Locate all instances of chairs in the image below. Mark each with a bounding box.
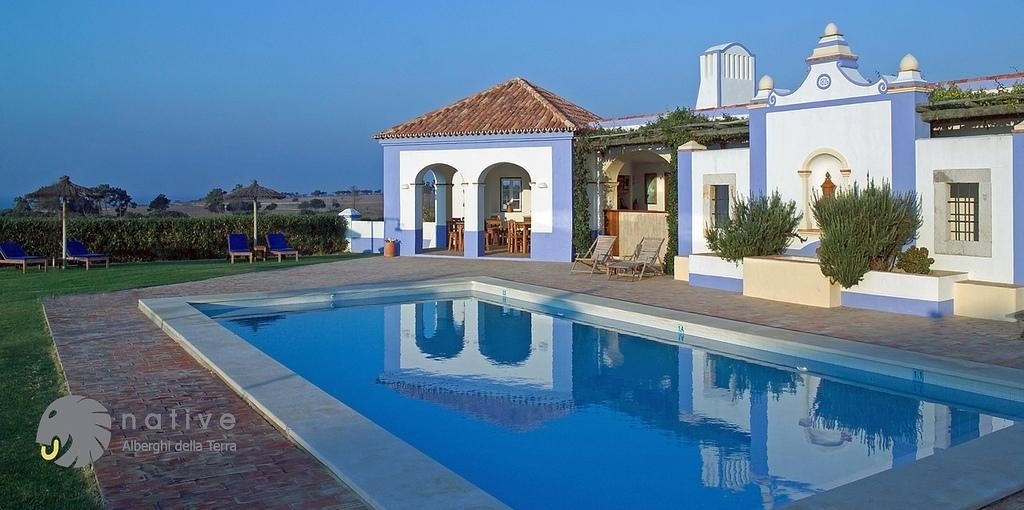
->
[607,236,666,282]
[264,232,299,263]
[568,234,618,279]
[53,238,111,271]
[0,242,51,275]
[226,233,253,264]
[447,216,530,255]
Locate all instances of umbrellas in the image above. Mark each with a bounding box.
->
[25,176,105,271]
[220,180,287,245]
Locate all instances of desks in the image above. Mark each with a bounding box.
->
[254,246,266,262]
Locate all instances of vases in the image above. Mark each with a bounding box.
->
[508,204,513,212]
[384,243,395,256]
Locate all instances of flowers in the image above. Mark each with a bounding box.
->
[385,237,397,242]
